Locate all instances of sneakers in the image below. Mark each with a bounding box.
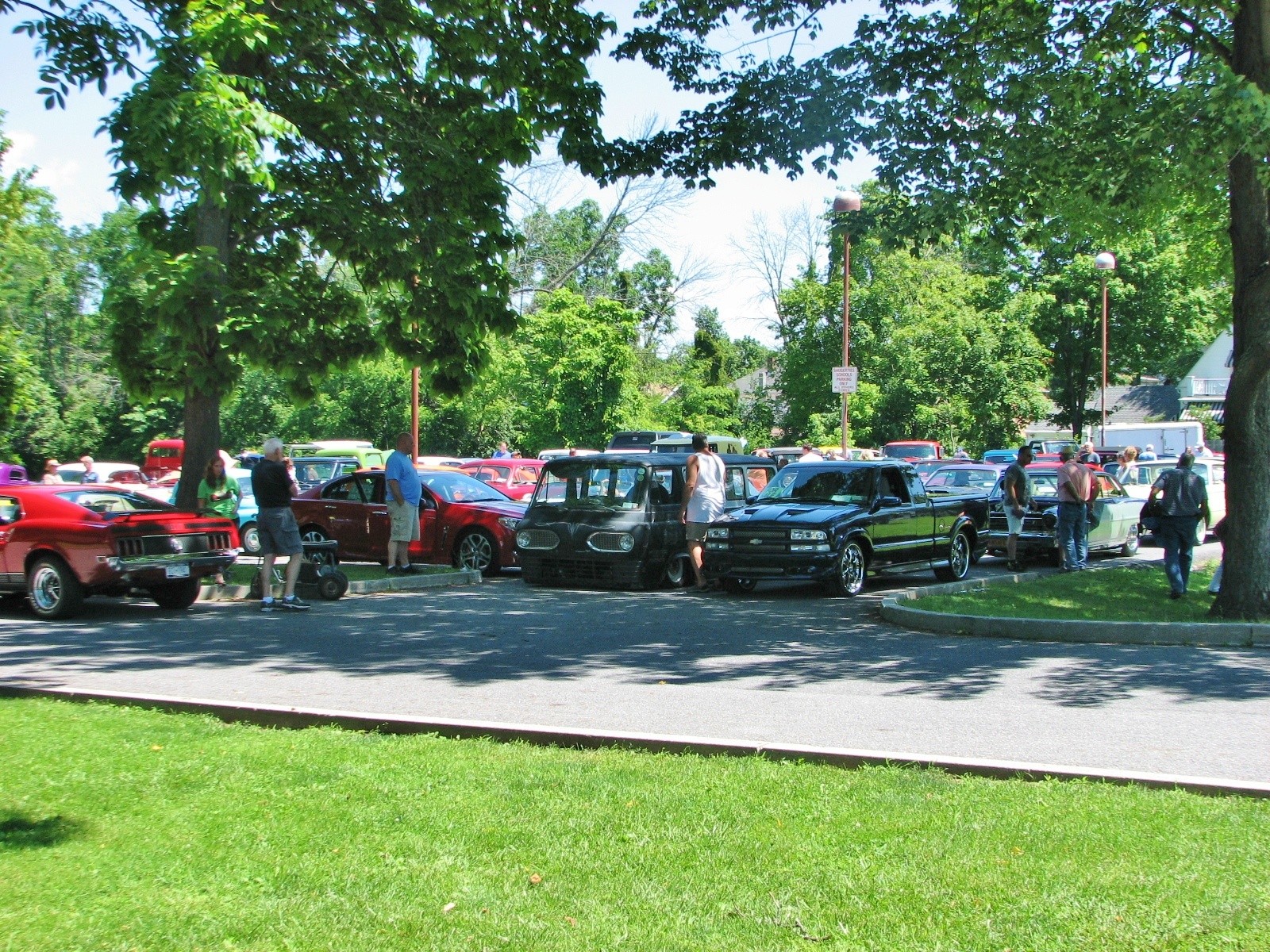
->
[214,579,226,584]
[259,598,285,612]
[1008,560,1026,572]
[282,595,310,608]
[687,584,709,593]
[401,562,423,573]
[387,566,405,576]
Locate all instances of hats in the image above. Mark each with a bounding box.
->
[1193,441,1205,448]
[1059,446,1075,454]
[45,459,62,470]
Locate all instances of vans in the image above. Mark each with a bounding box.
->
[517,452,779,591]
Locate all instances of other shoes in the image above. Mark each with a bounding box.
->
[1059,567,1071,573]
[1208,590,1220,596]
[1171,592,1181,599]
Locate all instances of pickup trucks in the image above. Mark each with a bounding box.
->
[702,459,990,600]
[880,440,962,488]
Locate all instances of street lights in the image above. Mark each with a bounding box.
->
[1095,252,1117,447]
[831,190,863,462]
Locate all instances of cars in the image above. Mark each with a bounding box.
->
[1115,455,1228,544]
[986,467,1151,566]
[923,463,1014,498]
[142,439,188,479]
[0,483,246,622]
[56,461,147,485]
[299,467,528,578]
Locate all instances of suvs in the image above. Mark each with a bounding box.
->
[0,428,877,557]
[979,439,1142,468]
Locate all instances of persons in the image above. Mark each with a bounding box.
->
[1138,444,1158,461]
[1184,446,1194,456]
[1116,446,1138,484]
[1149,451,1207,599]
[1075,442,1101,468]
[307,464,318,480]
[1004,445,1037,573]
[195,456,244,587]
[42,460,63,485]
[678,432,728,593]
[510,452,520,459]
[77,456,101,483]
[1208,515,1226,595]
[491,442,511,460]
[385,432,422,577]
[746,443,877,495]
[1193,441,1214,459]
[1056,445,1101,572]
[251,438,311,613]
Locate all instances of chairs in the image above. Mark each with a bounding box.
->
[627,469,667,508]
[865,476,894,507]
[334,490,348,499]
[810,477,833,501]
